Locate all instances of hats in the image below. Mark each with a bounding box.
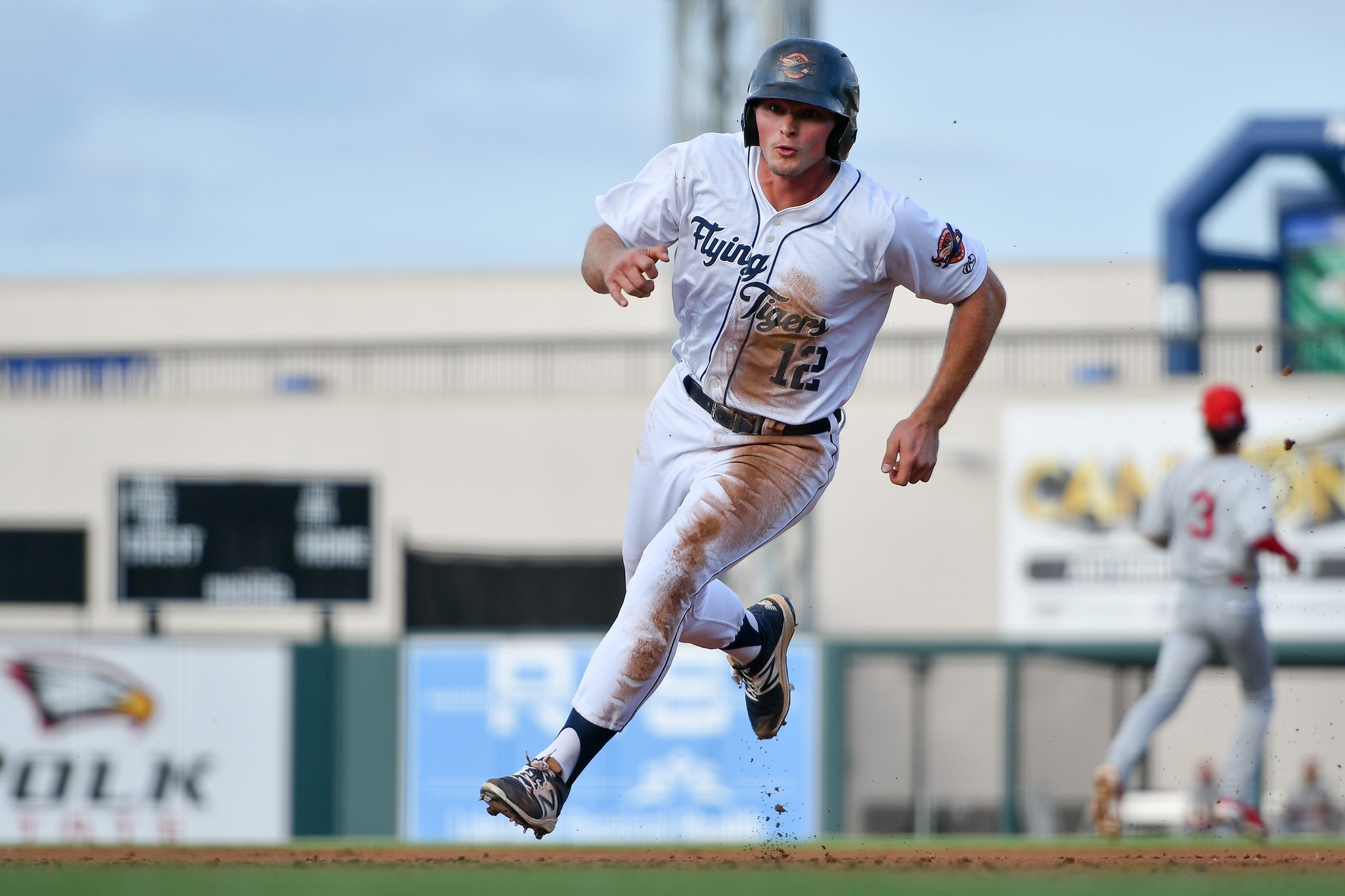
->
[1203,386,1243,429]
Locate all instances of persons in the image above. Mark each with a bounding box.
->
[1090,384,1299,846]
[1282,762,1336,833]
[479,36,1007,840]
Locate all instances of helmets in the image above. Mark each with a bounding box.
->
[741,37,860,162]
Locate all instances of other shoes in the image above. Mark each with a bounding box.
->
[1211,797,1266,843]
[1094,762,1124,836]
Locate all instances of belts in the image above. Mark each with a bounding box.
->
[682,375,842,436]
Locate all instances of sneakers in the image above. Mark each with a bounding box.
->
[730,595,798,738]
[479,748,567,840]
